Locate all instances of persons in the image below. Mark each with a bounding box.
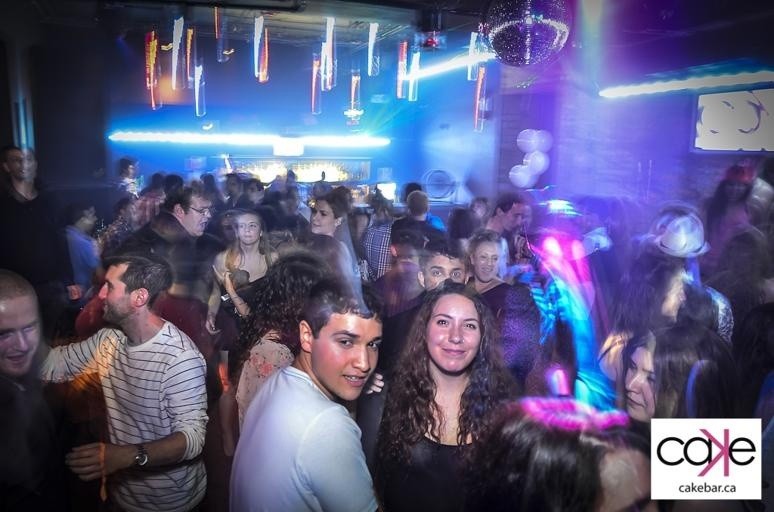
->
[0,147,772,512]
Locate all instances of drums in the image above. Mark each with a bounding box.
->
[421,169,453,199]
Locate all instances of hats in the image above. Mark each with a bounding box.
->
[653,204,712,258]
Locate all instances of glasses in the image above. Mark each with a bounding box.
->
[188,206,218,216]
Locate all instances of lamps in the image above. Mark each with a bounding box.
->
[485,1,575,69]
